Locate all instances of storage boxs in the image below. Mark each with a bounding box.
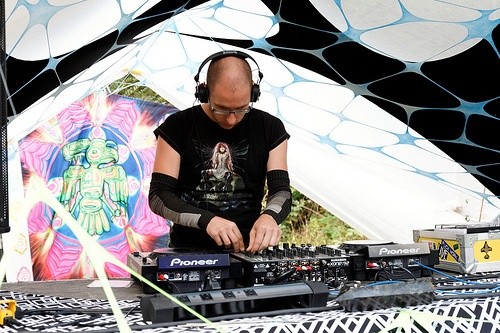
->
[413,224,500,273]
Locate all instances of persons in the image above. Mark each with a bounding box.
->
[148,57,292,252]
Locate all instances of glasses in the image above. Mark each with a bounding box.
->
[208,98,253,115]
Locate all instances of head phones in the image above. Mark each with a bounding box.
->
[193,50,263,104]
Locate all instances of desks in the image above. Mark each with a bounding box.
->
[0,270,500,333]
